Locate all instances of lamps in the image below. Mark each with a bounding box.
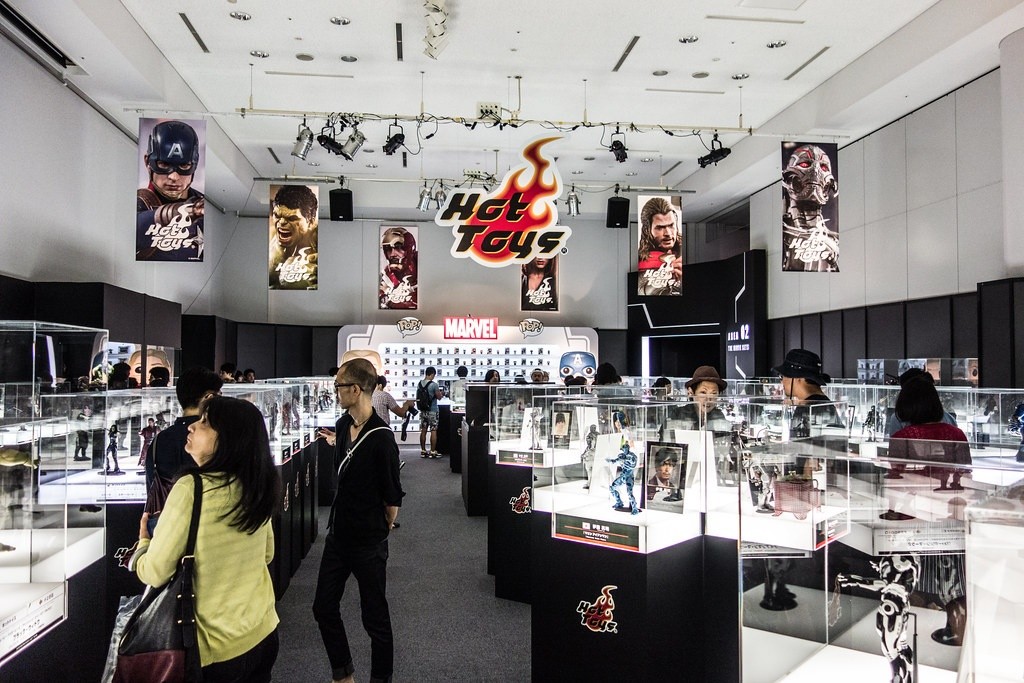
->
[292,115,731,216]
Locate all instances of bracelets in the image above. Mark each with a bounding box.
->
[332,438,335,445]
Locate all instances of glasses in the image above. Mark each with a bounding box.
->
[334,381,365,392]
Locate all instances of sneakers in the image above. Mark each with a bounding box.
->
[421,449,430,457]
[429,451,442,458]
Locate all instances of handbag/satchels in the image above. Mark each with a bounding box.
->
[142,475,175,538]
[100,470,206,683]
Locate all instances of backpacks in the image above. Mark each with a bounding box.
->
[416,380,436,410]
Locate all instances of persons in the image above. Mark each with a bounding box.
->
[0,407,166,553]
[416,367,445,458]
[591,362,621,392]
[651,378,671,400]
[451,366,469,403]
[129,396,282,683]
[138,121,204,262]
[563,375,589,393]
[605,444,639,516]
[381,228,416,310]
[521,258,557,311]
[555,414,567,434]
[771,349,846,475]
[268,388,334,442]
[314,359,407,683]
[372,376,415,470]
[530,368,544,381]
[146,366,224,511]
[527,409,543,449]
[580,425,600,490]
[637,197,683,295]
[219,362,254,383]
[648,448,677,499]
[887,367,973,518]
[108,362,168,389]
[613,413,622,433]
[837,552,921,683]
[659,366,727,442]
[269,184,318,290]
[862,406,880,442]
[485,370,500,382]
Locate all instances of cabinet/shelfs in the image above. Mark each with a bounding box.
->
[0,321,1024,682]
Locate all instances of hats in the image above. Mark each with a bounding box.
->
[685,366,727,392]
[772,348,827,386]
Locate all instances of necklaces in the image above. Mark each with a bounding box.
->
[354,419,368,428]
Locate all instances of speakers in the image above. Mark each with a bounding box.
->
[329,189,354,221]
[607,198,629,229]
